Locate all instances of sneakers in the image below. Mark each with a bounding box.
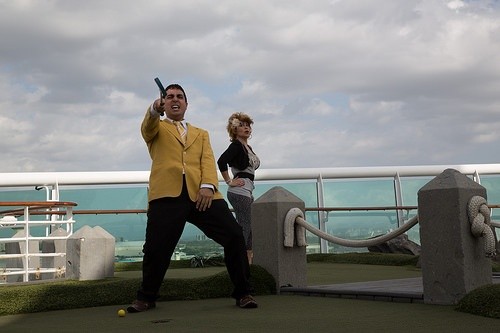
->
[236,293,257,307]
[127,299,154,312]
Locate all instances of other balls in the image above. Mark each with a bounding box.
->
[118,310,125,317]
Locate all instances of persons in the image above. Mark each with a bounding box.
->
[126,84,258,313]
[217,112,260,263]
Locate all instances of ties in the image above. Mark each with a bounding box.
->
[174,121,188,143]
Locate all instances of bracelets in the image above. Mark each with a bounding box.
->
[227,180,231,184]
